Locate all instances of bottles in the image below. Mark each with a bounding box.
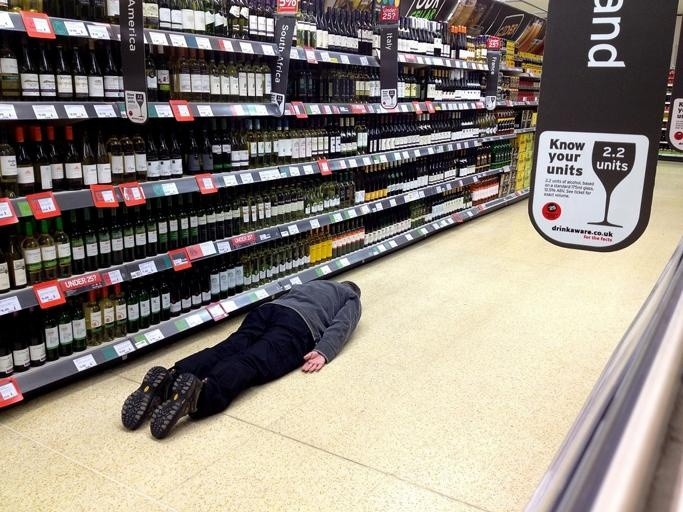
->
[0,0,517,377]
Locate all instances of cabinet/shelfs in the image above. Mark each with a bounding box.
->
[0,0,541,401]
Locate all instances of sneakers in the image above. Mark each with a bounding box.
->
[121,365,171,430]
[150,373,203,439]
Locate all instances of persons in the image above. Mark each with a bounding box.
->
[122,280,364,438]
